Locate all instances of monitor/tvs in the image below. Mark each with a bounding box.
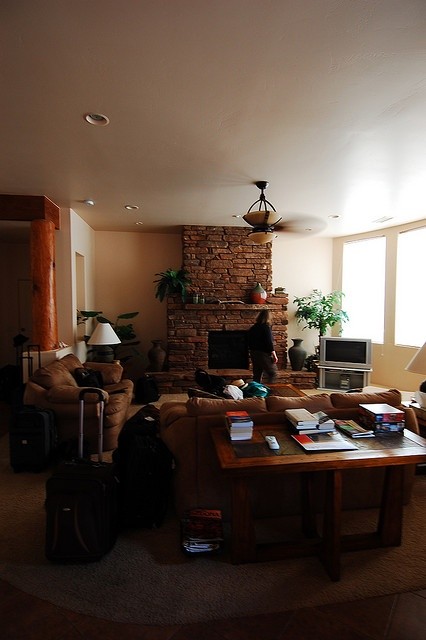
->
[320,336,372,369]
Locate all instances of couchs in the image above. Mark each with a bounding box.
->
[155,390,420,519]
[23,353,134,451]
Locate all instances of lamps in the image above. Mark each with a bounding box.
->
[87,322,122,362]
[243,181,279,245]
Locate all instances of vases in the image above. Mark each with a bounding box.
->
[148,339,167,369]
[252,282,268,303]
[289,339,307,371]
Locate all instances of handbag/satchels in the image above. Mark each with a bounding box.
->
[134,373,161,402]
[194,368,231,398]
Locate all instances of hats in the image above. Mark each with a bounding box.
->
[12,333,29,347]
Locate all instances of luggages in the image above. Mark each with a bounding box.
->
[44,387,119,565]
[0,364,24,404]
[9,357,55,474]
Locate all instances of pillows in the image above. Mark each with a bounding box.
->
[187,388,222,399]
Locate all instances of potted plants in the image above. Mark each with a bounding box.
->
[154,268,192,302]
[295,290,349,371]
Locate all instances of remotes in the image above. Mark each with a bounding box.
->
[265,435,280,450]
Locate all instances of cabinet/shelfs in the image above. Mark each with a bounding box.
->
[316,366,370,393]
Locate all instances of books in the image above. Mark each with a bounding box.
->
[335,403,405,438]
[224,410,254,441]
[285,408,335,435]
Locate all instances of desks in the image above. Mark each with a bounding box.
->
[207,426,425,582]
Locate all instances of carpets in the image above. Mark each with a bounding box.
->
[1,439,425,626]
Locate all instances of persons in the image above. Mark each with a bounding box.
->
[249,310,278,384]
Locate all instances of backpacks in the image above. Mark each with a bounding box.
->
[112,404,177,538]
